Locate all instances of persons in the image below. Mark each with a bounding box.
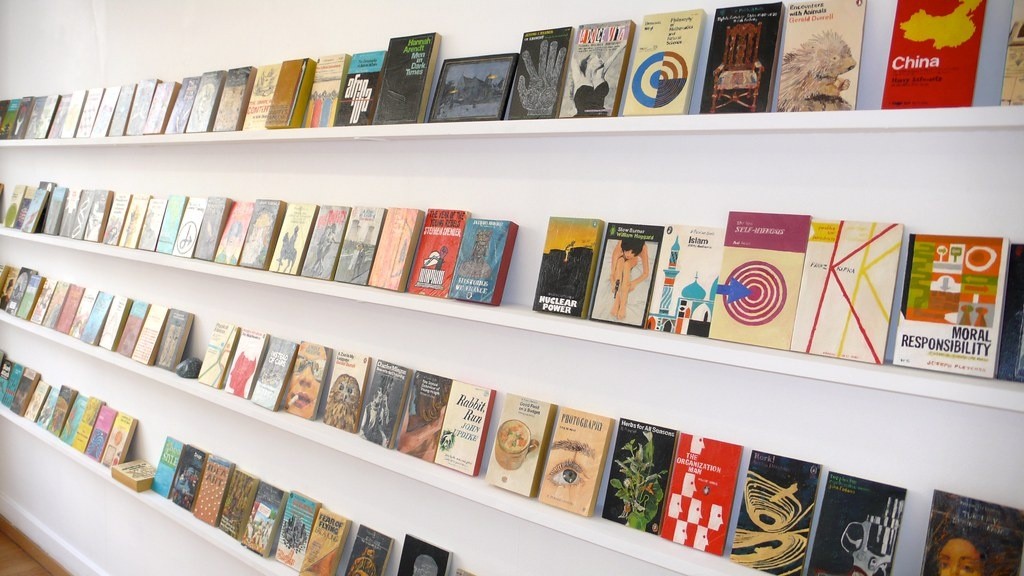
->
[397,371,453,463]
[937,530,996,576]
[285,342,326,419]
[312,223,339,275]
[610,238,649,319]
[353,245,365,277]
[360,376,389,448]
[570,40,626,117]
[0,279,13,311]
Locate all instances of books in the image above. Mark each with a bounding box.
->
[0,181,1024,576]
[0,0,1024,140]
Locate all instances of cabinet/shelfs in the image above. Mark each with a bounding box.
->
[0,105,1024,575]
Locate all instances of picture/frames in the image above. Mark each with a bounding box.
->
[426,52,520,122]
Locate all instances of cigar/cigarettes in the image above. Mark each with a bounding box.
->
[285,396,299,409]
